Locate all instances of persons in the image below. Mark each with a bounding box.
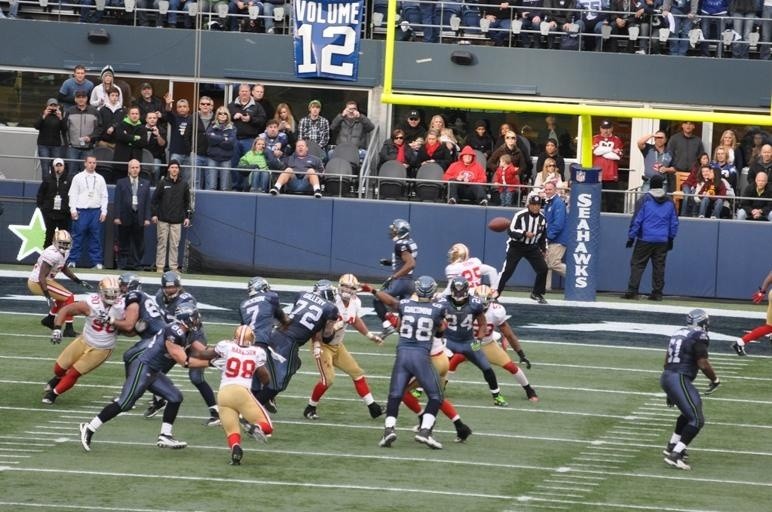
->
[3,0,772,469]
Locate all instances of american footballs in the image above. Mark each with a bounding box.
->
[488,217,511,232]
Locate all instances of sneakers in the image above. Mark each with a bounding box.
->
[42,376,61,404]
[208,396,278,444]
[229,444,243,464]
[370,405,387,419]
[662,442,692,471]
[379,426,396,447]
[144,399,168,419]
[78,420,96,453]
[493,394,509,407]
[618,291,664,301]
[303,407,319,419]
[156,434,188,450]
[448,197,456,204]
[528,291,547,305]
[527,395,539,402]
[313,189,322,197]
[729,340,749,356]
[270,186,281,195]
[41,316,81,337]
[411,389,422,398]
[480,199,488,206]
[415,409,471,448]
[67,261,182,276]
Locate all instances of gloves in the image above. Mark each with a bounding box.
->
[311,348,323,361]
[51,326,64,345]
[752,286,766,304]
[470,338,482,353]
[377,258,390,269]
[380,276,392,292]
[666,396,675,408]
[47,296,58,308]
[358,282,374,293]
[518,357,531,369]
[668,239,673,250]
[370,335,383,346]
[97,309,116,326]
[625,237,634,248]
[703,377,720,395]
[80,280,94,289]
[209,356,227,372]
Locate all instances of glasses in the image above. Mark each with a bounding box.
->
[200,102,212,106]
[218,112,228,116]
[394,136,404,140]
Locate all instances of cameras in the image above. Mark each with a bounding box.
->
[51,109,56,112]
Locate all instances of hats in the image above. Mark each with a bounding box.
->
[101,65,115,78]
[76,89,88,98]
[407,110,420,120]
[53,158,65,167]
[47,98,58,107]
[528,195,542,205]
[598,119,613,130]
[307,100,322,109]
[650,175,665,184]
[141,82,152,90]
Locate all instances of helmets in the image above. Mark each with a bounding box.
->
[97,273,143,305]
[234,325,256,347]
[248,276,271,297]
[450,276,492,311]
[162,271,182,300]
[414,275,438,298]
[175,302,202,334]
[684,308,711,332]
[53,229,73,255]
[389,218,411,240]
[314,273,360,302]
[446,243,469,264]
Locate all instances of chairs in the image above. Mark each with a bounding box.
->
[302,136,530,207]
[93,146,154,186]
[674,172,689,217]
